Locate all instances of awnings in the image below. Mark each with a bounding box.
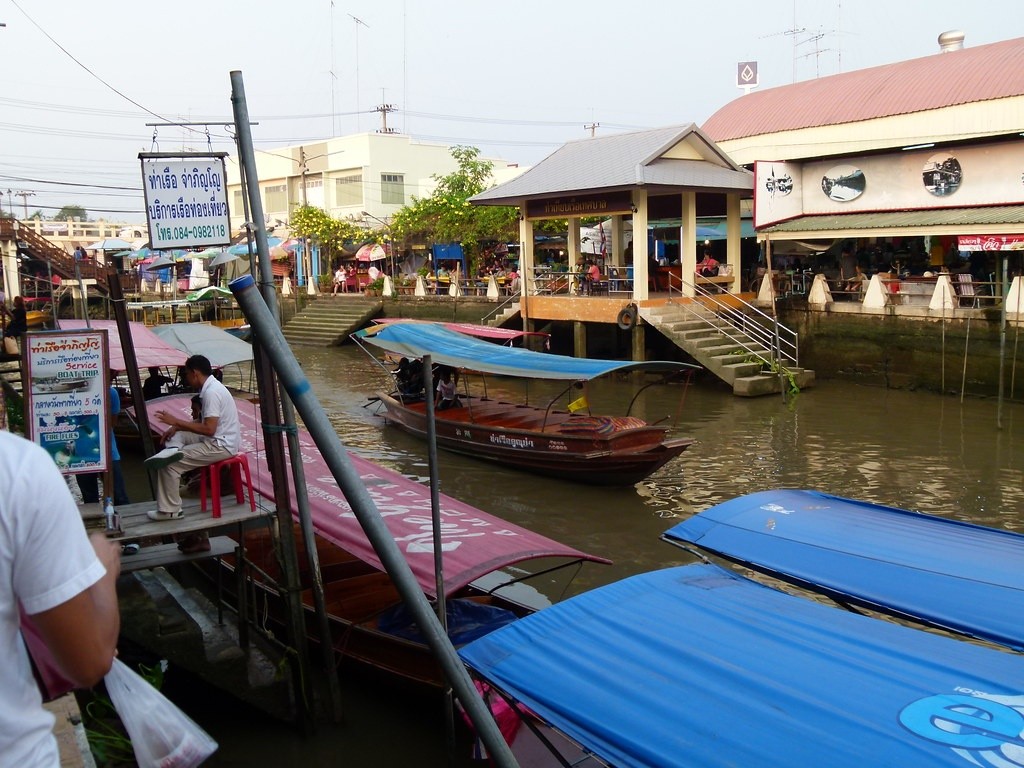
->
[681,218,757,240]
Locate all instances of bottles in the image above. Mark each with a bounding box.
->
[105,497,115,530]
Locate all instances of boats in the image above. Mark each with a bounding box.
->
[347,316,701,488]
[661,487,1024,656]
[204,317,252,341]
[0,297,53,328]
[463,563,1023,767]
[56,318,258,395]
[132,392,618,695]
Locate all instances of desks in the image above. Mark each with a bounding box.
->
[483,277,512,284]
[905,275,954,282]
[430,276,451,283]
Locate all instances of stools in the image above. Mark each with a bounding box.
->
[201,451,256,518]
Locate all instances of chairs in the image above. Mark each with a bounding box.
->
[954,274,981,309]
[569,265,657,299]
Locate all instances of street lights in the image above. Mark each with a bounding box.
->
[0,189,13,217]
[15,189,37,220]
[274,218,308,291]
[362,210,396,292]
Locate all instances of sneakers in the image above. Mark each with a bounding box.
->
[144,448,184,469]
[146,508,185,521]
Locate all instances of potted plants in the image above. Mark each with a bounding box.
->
[277,256,291,276]
[319,274,334,293]
[364,275,417,296]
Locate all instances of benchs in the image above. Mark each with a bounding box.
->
[407,399,585,433]
[218,524,493,630]
[697,276,735,294]
[212,318,244,328]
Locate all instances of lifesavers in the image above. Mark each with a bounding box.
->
[626,303,637,315]
[618,308,636,330]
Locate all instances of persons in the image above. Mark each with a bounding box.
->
[333,260,519,296]
[0,429,122,768]
[575,240,720,295]
[0,296,28,344]
[390,357,463,411]
[74,246,87,260]
[144,355,240,520]
[792,250,868,302]
[75,369,130,506]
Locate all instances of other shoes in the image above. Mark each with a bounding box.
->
[178,530,210,554]
[455,399,463,408]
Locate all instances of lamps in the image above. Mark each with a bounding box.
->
[630,201,637,213]
[516,211,523,221]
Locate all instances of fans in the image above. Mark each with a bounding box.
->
[890,255,906,276]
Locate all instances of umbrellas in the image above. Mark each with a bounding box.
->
[84,236,298,271]
[356,243,395,273]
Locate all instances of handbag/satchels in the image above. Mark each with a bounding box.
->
[101,658,219,768]
